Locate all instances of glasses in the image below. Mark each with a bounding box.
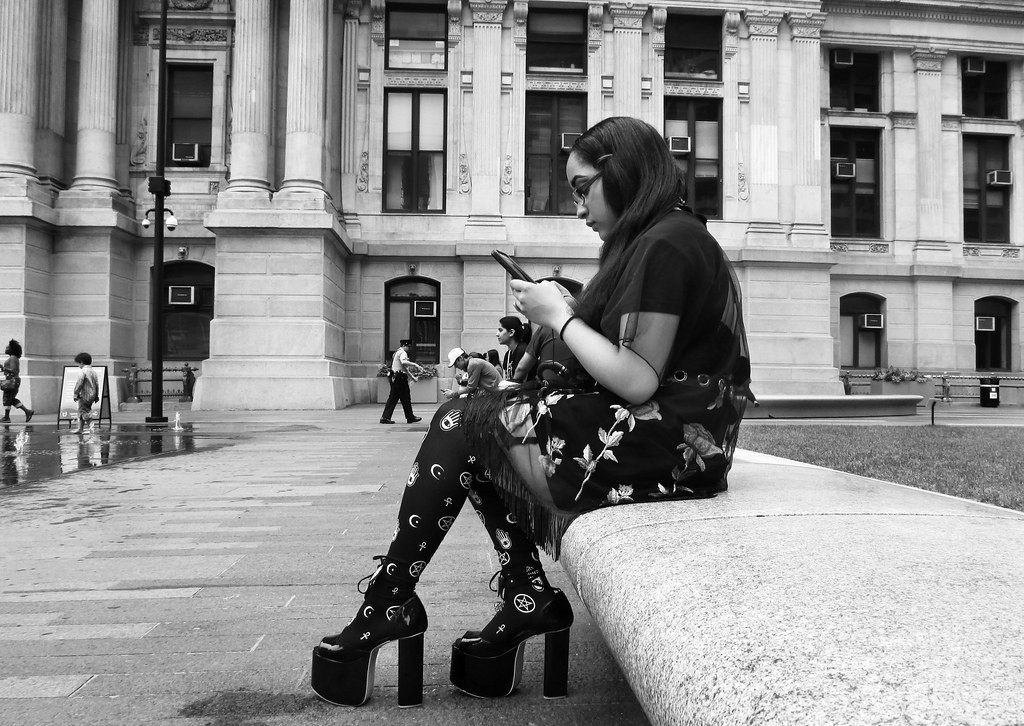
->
[571,169,604,207]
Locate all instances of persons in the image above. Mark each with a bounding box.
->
[442,347,503,399]
[73,352,100,435]
[0,339,35,423]
[380,339,423,424]
[513,281,584,385]
[311,116,759,709]
[496,316,532,383]
[456,352,479,398]
[487,350,503,379]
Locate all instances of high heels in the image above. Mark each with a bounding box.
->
[310,568,428,710]
[449,567,574,699]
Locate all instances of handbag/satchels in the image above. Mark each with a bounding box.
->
[0,370,21,391]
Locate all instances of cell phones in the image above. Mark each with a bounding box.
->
[440,389,445,392]
[491,249,535,285]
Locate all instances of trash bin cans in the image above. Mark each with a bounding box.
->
[980,378,999,407]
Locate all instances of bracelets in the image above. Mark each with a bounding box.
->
[560,316,575,340]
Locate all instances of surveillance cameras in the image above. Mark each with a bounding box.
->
[142,219,151,229]
[166,216,178,231]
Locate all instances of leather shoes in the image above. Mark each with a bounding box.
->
[380,415,395,424]
[407,416,423,423]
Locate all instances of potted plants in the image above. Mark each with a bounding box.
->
[871,365,936,406]
[377,361,438,404]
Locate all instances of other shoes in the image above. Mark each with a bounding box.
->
[0,416,11,423]
[26,409,34,422]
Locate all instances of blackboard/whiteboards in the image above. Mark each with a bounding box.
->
[59,366,111,420]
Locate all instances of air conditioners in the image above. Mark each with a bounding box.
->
[666,136,692,156]
[414,300,438,317]
[558,132,584,154]
[172,143,203,162]
[975,316,995,332]
[858,314,884,329]
[986,170,1013,187]
[834,49,853,68]
[832,163,856,180]
[168,285,201,305]
[963,57,986,77]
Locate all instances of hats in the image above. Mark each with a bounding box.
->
[399,339,413,347]
[447,346,463,368]
